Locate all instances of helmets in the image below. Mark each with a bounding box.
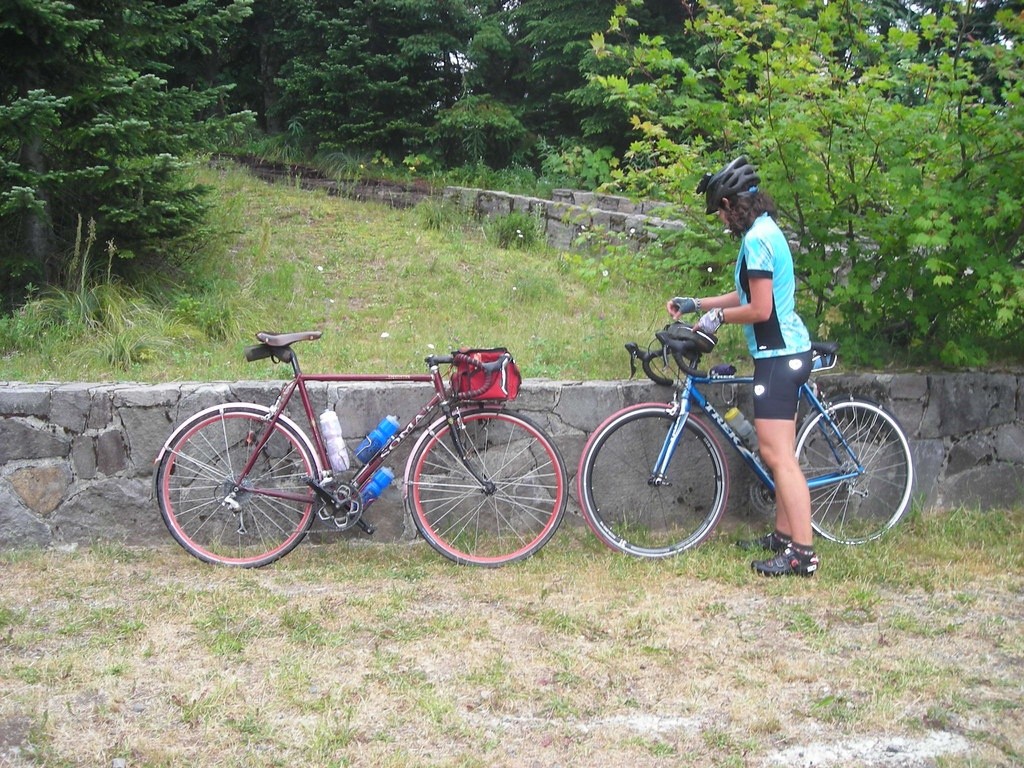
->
[706,155,762,215]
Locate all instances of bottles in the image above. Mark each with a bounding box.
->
[349,466,396,515]
[319,408,350,473]
[354,414,401,462]
[725,407,758,452]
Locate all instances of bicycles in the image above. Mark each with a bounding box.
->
[154,330,570,570]
[575,331,915,559]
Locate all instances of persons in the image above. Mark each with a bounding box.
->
[666,155,818,579]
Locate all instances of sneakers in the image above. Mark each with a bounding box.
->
[751,542,817,578]
[734,530,794,555]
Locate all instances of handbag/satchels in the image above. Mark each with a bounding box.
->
[448,349,520,401]
